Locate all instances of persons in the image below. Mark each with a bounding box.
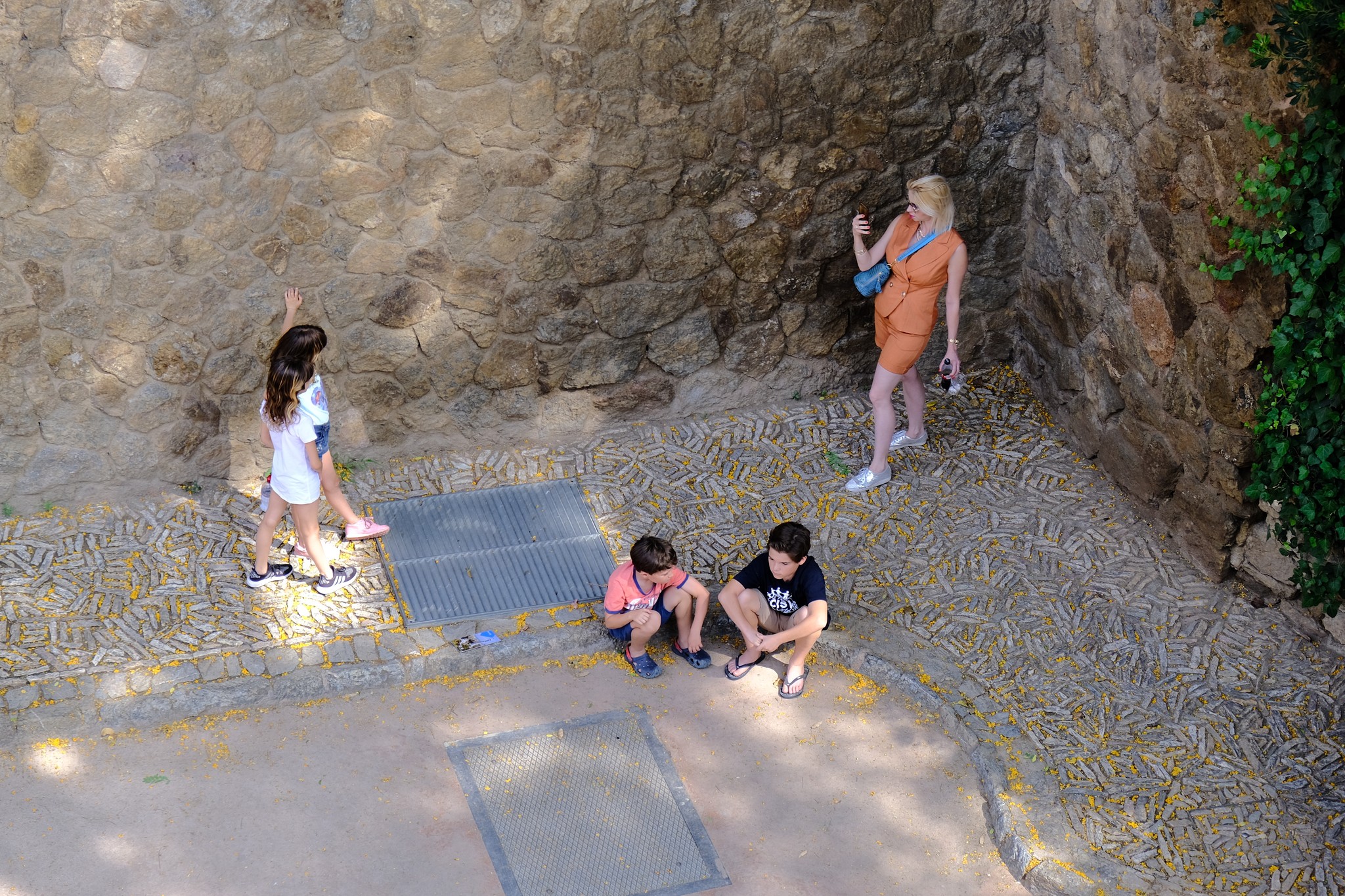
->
[263,287,389,559]
[845,175,969,492]
[247,356,357,594]
[604,535,711,678]
[718,521,831,699]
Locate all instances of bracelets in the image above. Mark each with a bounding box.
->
[853,243,866,255]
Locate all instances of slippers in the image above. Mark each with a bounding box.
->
[671,638,711,669]
[624,643,662,678]
[725,651,765,680]
[779,665,809,699]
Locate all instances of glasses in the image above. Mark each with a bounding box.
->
[905,194,920,214]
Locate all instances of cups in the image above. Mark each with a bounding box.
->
[949,372,967,395]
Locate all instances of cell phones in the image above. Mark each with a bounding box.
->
[859,202,869,235]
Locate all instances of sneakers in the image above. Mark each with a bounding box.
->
[888,430,929,450]
[345,516,390,540]
[844,465,892,492]
[294,540,312,559]
[246,562,293,587]
[316,565,357,594]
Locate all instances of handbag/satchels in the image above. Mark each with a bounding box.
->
[853,261,892,298]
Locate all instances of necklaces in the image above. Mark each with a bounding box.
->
[916,225,932,244]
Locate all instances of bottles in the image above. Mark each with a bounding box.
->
[259,475,272,512]
[941,358,953,391]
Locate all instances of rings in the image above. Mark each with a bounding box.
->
[856,226,858,229]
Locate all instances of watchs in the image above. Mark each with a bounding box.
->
[947,339,959,345]
